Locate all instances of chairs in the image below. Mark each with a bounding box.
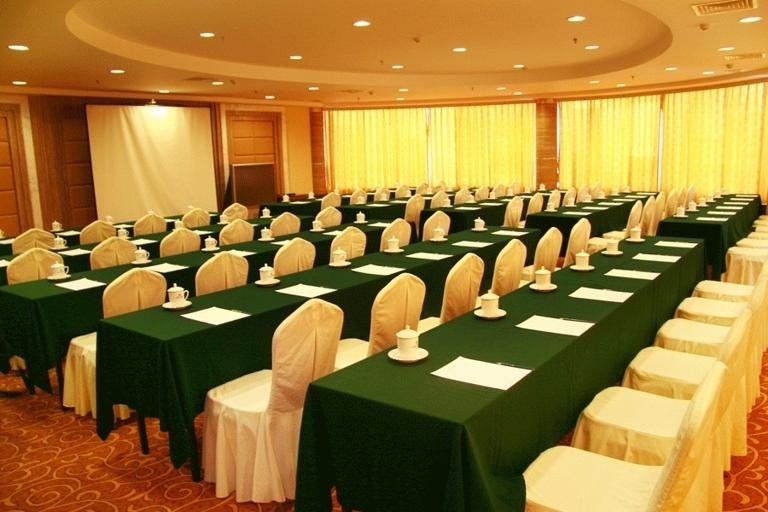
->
[0,181,768,512]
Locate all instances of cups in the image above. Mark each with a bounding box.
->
[534,265,552,288]
[606,238,620,252]
[479,290,501,314]
[395,325,420,357]
[167,282,189,305]
[575,248,590,268]
[442,182,728,217]
[630,226,642,240]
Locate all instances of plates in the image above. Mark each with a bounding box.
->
[625,237,647,243]
[568,264,596,272]
[531,282,557,292]
[254,279,280,286]
[602,250,625,256]
[388,348,430,364]
[162,300,192,310]
[474,309,509,320]
